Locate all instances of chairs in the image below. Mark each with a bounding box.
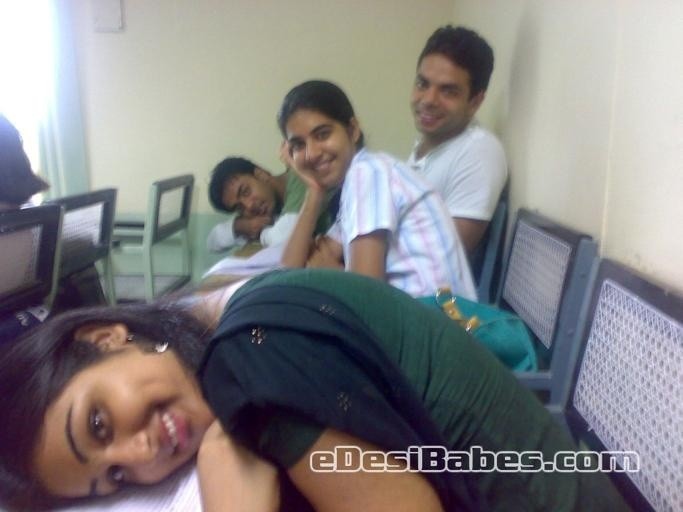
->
[477,209,598,405]
[1,206,72,342]
[111,174,194,306]
[471,199,506,305]
[514,259,683,512]
[48,188,116,314]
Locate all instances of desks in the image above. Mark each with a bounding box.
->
[0,238,264,512]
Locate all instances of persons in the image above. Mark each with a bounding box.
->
[0,116,78,346]
[206,157,337,248]
[0,264,584,512]
[276,79,479,304]
[405,23,508,265]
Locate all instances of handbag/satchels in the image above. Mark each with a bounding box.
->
[415,287,538,373]
